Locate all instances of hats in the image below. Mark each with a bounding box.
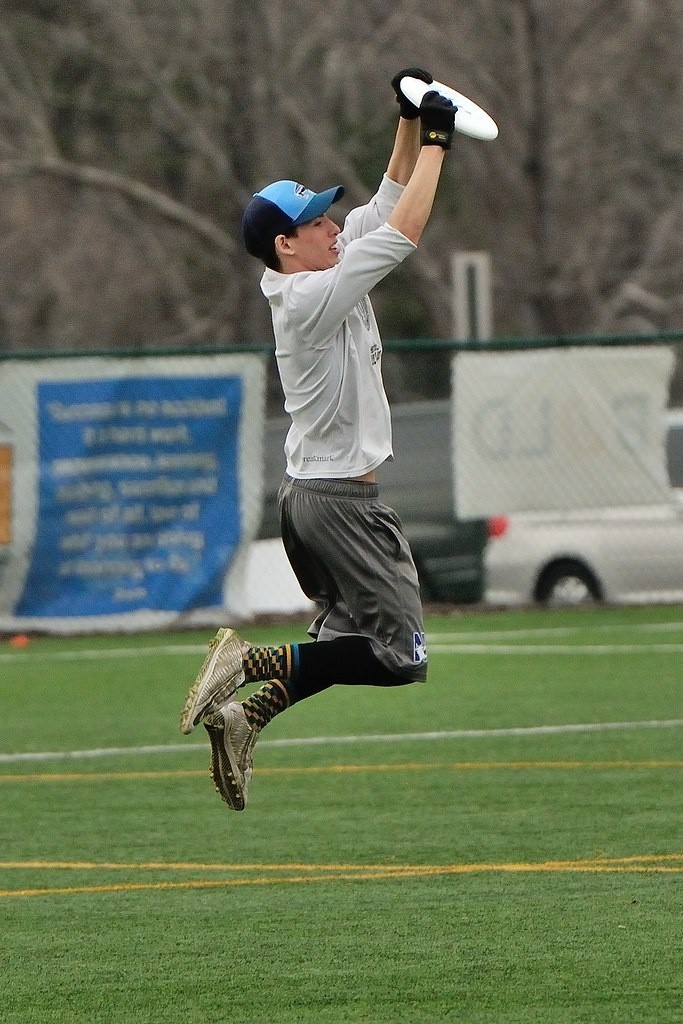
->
[242,179,344,258]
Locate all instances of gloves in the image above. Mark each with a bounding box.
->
[419,90,458,150]
[391,67,434,120]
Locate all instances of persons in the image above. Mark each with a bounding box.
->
[177,69,458,811]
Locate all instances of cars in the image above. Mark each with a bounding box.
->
[255,484,509,606]
[481,406,683,608]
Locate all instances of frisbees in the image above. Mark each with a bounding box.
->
[399,75,500,142]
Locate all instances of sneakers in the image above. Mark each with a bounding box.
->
[203,700,259,811]
[180,627,252,734]
